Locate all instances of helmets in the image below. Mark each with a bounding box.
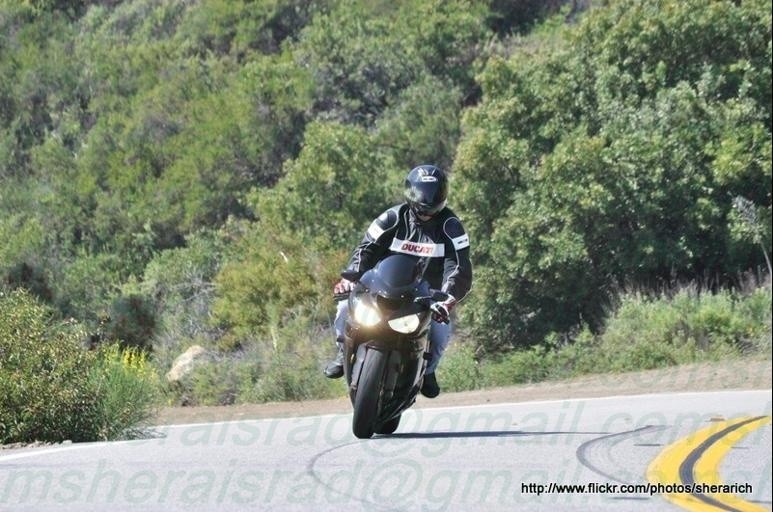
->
[404,165,448,218]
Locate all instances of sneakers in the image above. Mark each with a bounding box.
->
[324,363,344,379]
[421,372,440,398]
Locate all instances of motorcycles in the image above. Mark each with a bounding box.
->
[333,255,450,439]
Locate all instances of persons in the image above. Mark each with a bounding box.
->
[323,164,472,397]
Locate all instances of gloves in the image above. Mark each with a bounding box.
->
[333,278,355,294]
[430,294,457,324]
